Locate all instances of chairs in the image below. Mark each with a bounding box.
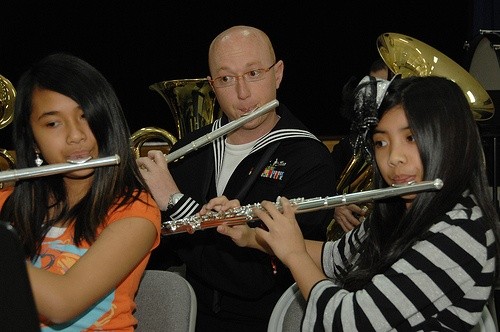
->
[268,280,496,332]
[132,270,197,332]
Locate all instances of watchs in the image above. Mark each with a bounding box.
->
[167,193,184,209]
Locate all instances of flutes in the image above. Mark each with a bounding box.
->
[160,178,445,233]
[141,100,278,174]
[0,154,121,183]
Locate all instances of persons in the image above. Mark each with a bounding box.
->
[328,53,394,234]
[0,54,161,332]
[135,26,336,332]
[199,75,500,332]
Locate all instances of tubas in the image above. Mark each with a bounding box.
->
[325,32,496,240]
[126,77,217,190]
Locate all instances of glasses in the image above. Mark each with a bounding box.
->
[210,61,277,89]
